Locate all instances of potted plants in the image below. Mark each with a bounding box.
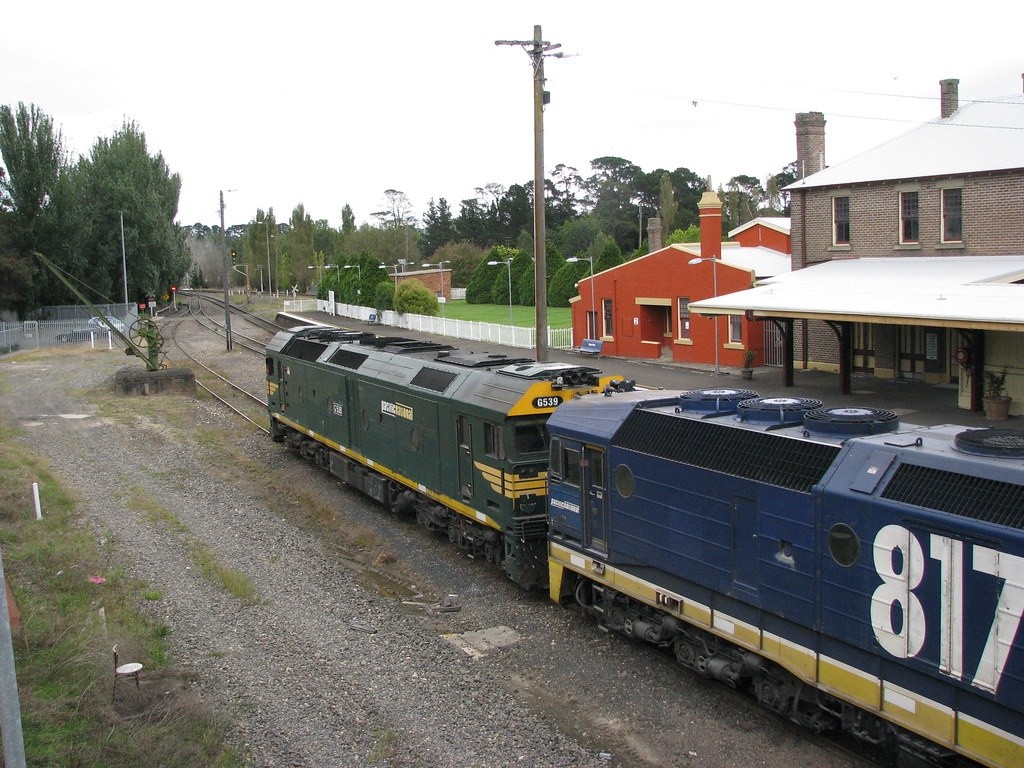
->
[741,348,756,379]
[982,365,1012,421]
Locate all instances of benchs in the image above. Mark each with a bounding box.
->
[363,314,376,326]
[574,338,603,360]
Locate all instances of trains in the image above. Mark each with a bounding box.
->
[263,323,1024,768]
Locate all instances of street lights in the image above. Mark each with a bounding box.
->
[378,265,398,293]
[487,256,513,325]
[565,256,596,341]
[118,210,128,304]
[307,265,322,289]
[344,265,360,281]
[421,261,451,318]
[688,255,722,375]
[325,265,340,283]
[257,222,271,297]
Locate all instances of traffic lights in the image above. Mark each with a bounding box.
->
[232,249,236,258]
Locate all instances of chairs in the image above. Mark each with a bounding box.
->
[111,644,143,703]
